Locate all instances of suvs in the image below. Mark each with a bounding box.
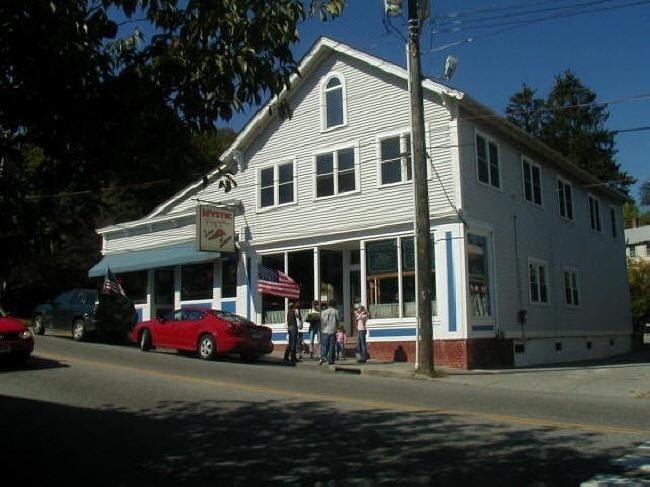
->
[32,288,138,343]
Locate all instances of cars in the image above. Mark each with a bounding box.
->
[128,308,273,360]
[0,306,35,359]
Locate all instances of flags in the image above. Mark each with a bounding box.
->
[257,261,301,300]
[103,267,125,295]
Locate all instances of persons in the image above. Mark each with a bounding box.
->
[319,301,327,310]
[306,301,321,358]
[319,300,340,364]
[354,307,371,362]
[336,325,347,360]
[284,299,301,362]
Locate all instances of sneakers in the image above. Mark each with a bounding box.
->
[283,357,304,363]
